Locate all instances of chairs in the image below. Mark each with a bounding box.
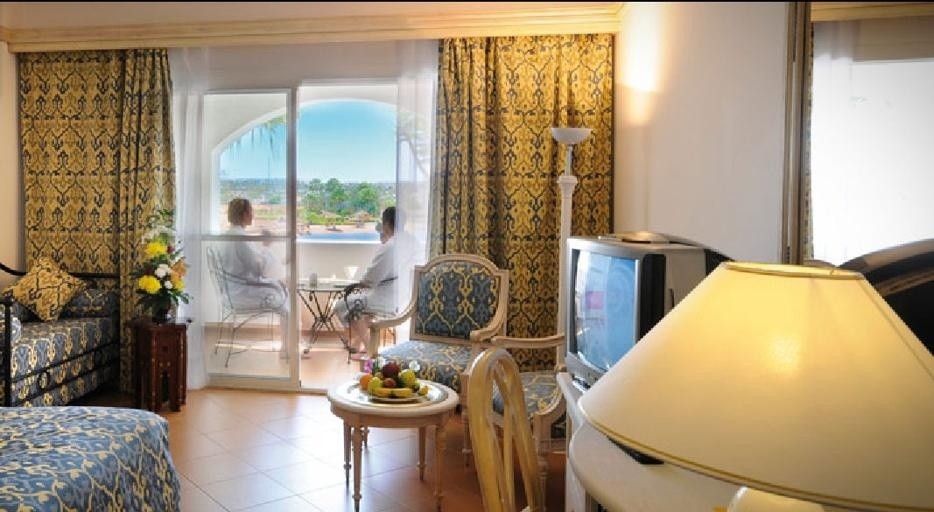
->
[467,349,543,512]
[367,253,510,467]
[344,275,399,365]
[491,332,567,512]
[205,246,291,367]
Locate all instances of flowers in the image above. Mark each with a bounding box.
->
[135,225,191,307]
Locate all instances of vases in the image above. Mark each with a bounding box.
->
[151,297,171,322]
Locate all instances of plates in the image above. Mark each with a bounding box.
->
[365,394,423,401]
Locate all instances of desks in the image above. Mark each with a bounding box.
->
[126,320,187,412]
[556,373,742,512]
[297,278,361,360]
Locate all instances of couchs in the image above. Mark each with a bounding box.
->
[0,262,119,407]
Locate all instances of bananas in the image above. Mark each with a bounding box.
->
[373,387,414,398]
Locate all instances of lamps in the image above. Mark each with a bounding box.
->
[549,127,592,370]
[577,261,934,512]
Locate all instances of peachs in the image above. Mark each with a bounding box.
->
[383,377,398,388]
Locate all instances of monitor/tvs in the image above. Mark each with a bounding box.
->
[564,230,735,465]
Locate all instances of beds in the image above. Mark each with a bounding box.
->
[0,403,182,512]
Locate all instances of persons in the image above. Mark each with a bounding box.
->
[333,207,418,357]
[216,198,312,354]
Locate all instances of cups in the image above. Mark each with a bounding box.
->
[376,223,386,234]
[343,265,357,278]
[309,272,317,288]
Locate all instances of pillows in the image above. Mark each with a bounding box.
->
[3,257,85,323]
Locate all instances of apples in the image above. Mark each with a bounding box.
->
[368,377,383,393]
[397,369,416,388]
[374,371,383,379]
[382,362,400,378]
